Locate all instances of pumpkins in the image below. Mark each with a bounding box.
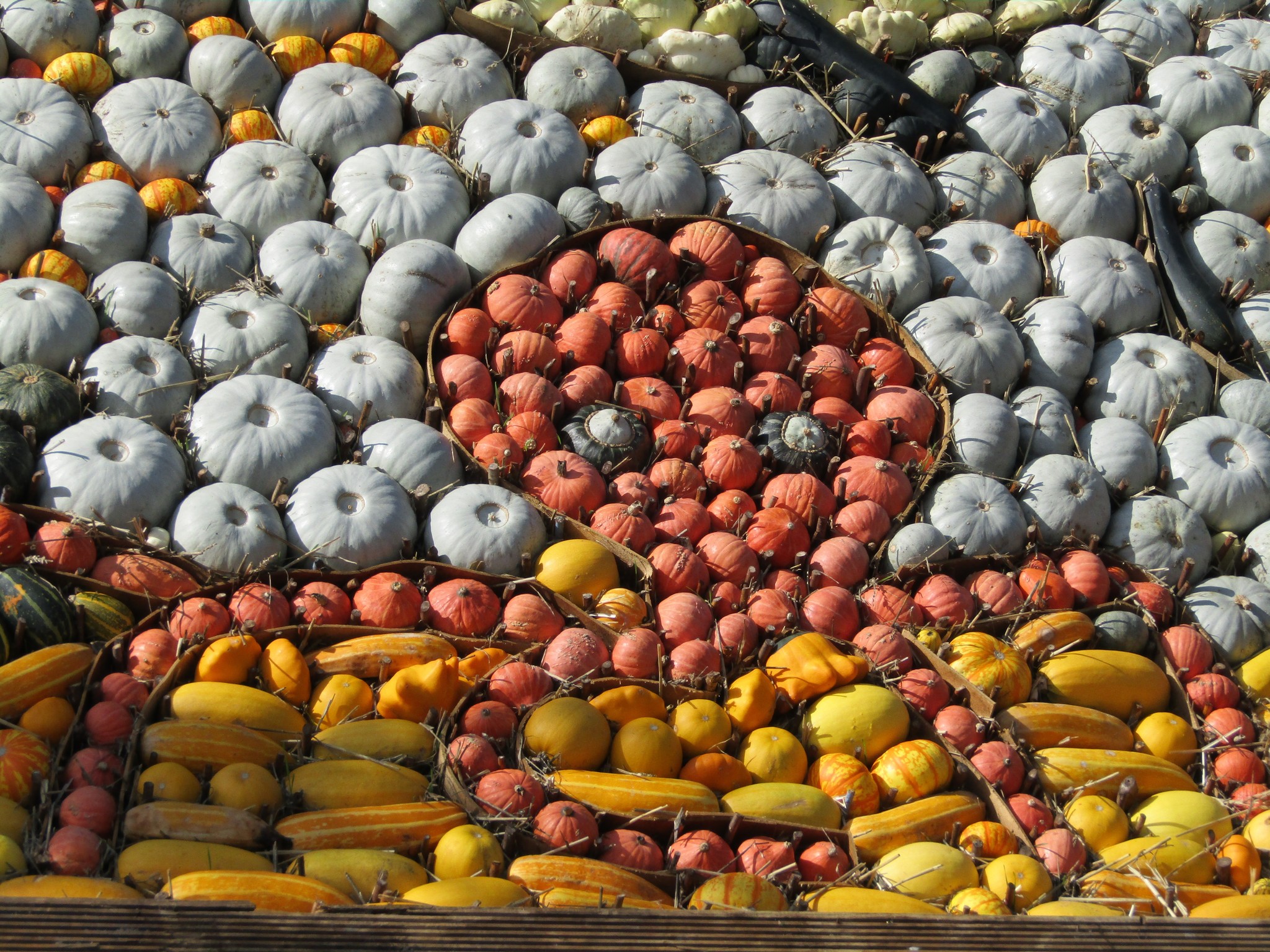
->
[0,0,1270,927]
